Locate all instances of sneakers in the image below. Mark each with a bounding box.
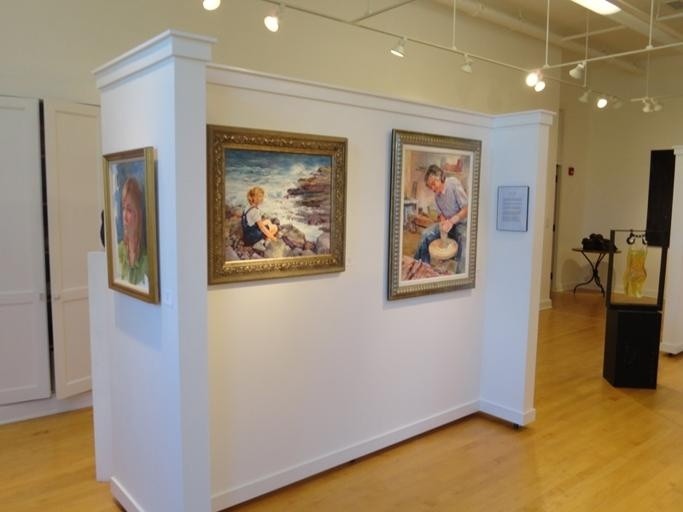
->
[252,240,270,252]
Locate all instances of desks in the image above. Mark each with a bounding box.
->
[570,246,622,299]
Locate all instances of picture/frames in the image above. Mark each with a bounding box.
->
[202,121,349,286]
[99,144,162,306]
[385,129,484,300]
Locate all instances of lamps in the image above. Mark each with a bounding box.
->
[264,3,283,35]
[525,69,546,94]
[641,98,663,113]
[574,1,622,16]
[580,91,623,110]
[462,59,474,76]
[568,63,586,81]
[391,37,408,60]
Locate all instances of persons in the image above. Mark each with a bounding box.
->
[413,164,468,274]
[241,186,278,251]
[117,178,149,286]
